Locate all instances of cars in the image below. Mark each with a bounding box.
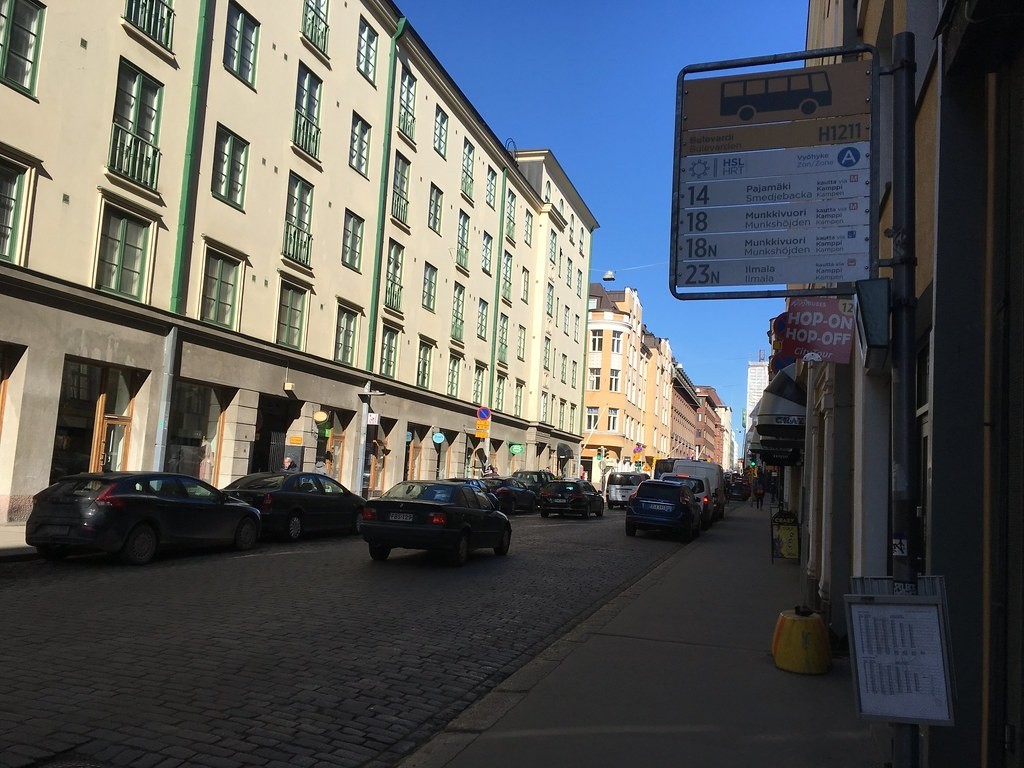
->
[479,477,537,514]
[625,480,703,542]
[360,479,513,562]
[658,471,719,531]
[441,477,501,510]
[24,471,260,565]
[724,469,751,502]
[218,470,370,541]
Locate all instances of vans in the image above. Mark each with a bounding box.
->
[606,472,651,509]
[512,470,558,500]
[672,459,729,523]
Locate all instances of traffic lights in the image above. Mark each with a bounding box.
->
[604,448,610,460]
[750,454,756,466]
[597,448,601,460]
[635,461,645,470]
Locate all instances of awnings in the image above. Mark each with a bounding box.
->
[748,364,807,468]
[557,443,574,459]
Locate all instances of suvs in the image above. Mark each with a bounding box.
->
[540,479,605,519]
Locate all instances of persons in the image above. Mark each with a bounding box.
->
[314,456,327,476]
[170,450,186,472]
[280,457,295,474]
[555,474,566,480]
[488,467,498,473]
[545,467,551,479]
[754,475,767,510]
